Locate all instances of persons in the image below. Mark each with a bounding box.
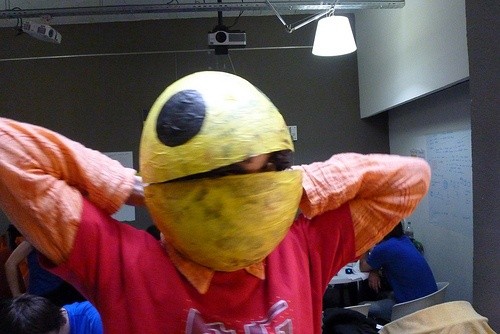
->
[360,221,438,324]
[0,70,431,334]
[5,237,71,305]
[0,293,102,334]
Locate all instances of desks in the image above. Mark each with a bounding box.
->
[329,260,371,308]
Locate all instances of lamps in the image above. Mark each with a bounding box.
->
[312,6,357,57]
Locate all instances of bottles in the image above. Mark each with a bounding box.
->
[405,222,413,242]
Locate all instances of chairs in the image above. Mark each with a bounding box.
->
[391,282,450,323]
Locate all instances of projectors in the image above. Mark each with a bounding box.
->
[22,21,61,45]
[208,30,247,54]
